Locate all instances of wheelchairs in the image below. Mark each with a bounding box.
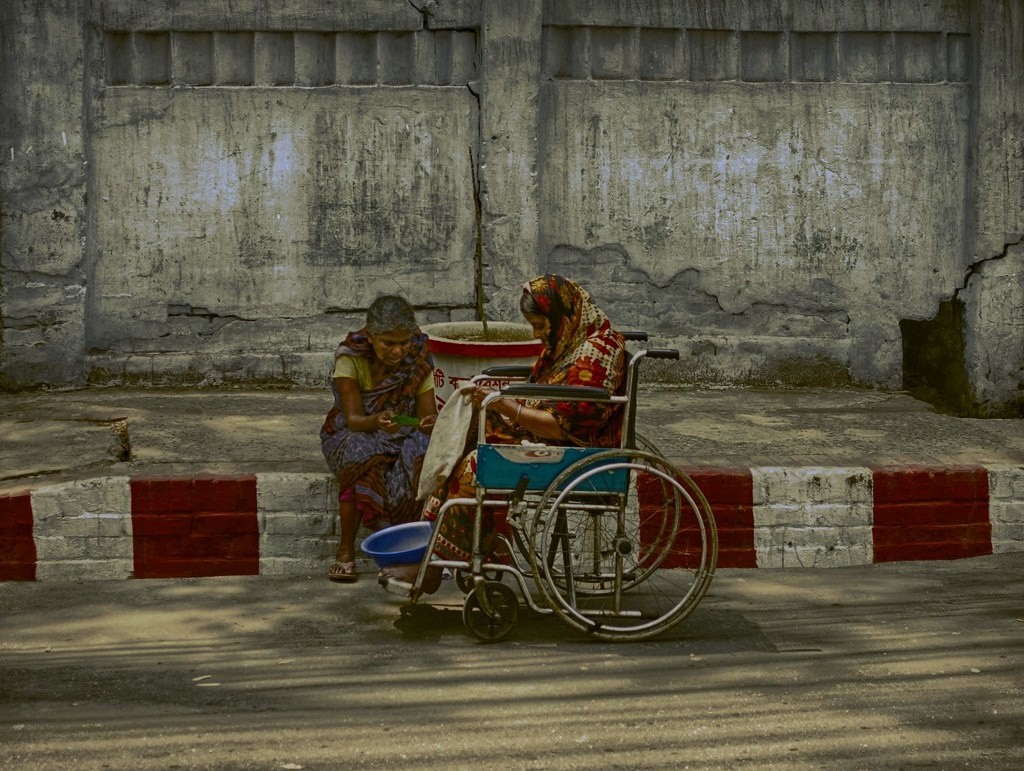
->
[379,332,720,643]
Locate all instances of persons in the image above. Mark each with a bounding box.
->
[377,274,625,595]
[319,296,452,579]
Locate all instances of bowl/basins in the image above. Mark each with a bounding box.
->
[361,521,436,570]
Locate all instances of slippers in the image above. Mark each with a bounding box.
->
[328,561,357,581]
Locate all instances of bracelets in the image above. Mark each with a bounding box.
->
[514,404,523,422]
